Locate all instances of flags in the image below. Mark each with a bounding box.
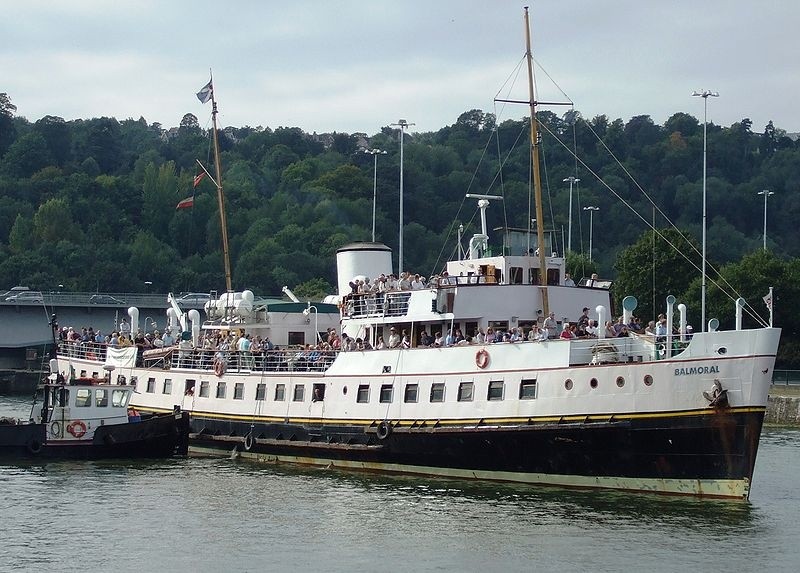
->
[176,196,193,209]
[196,80,213,104]
[194,161,207,188]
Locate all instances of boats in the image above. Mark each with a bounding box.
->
[35,359,190,461]
[45,3,784,505]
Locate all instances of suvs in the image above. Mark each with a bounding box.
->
[175,293,215,308]
[87,295,126,305]
[0,286,30,302]
[5,291,44,304]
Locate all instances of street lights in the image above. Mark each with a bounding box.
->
[757,189,775,255]
[365,147,390,243]
[582,206,599,264]
[562,176,580,261]
[690,88,720,332]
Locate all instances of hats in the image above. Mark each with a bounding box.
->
[583,308,590,312]
[390,327,396,331]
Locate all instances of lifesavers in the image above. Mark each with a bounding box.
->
[69,421,86,438]
[377,421,391,439]
[214,360,223,377]
[475,350,488,368]
[244,433,255,450]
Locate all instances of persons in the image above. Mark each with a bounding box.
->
[58,271,693,372]
